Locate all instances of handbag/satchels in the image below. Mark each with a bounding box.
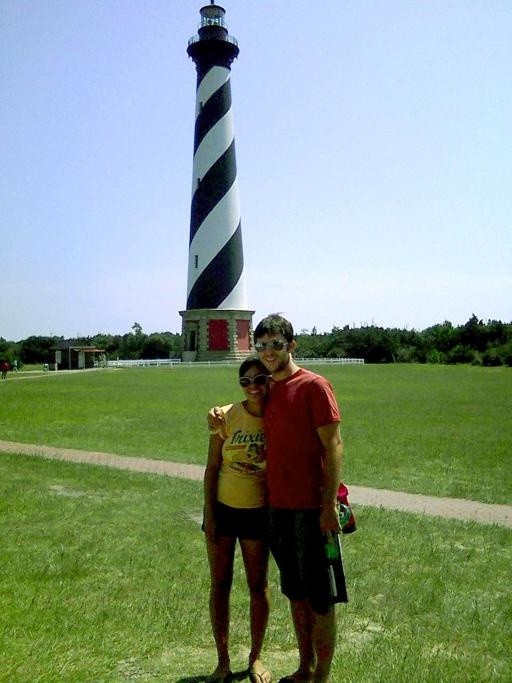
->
[337,483,356,534]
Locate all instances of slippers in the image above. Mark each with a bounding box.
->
[205,670,230,683]
[249,664,272,683]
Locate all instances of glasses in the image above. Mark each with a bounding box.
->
[239,374,269,388]
[254,342,288,351]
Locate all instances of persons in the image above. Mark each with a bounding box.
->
[90,351,107,368]
[206,311,350,683]
[196,354,273,682]
[1,359,9,379]
[42,360,50,371]
[11,357,18,375]
